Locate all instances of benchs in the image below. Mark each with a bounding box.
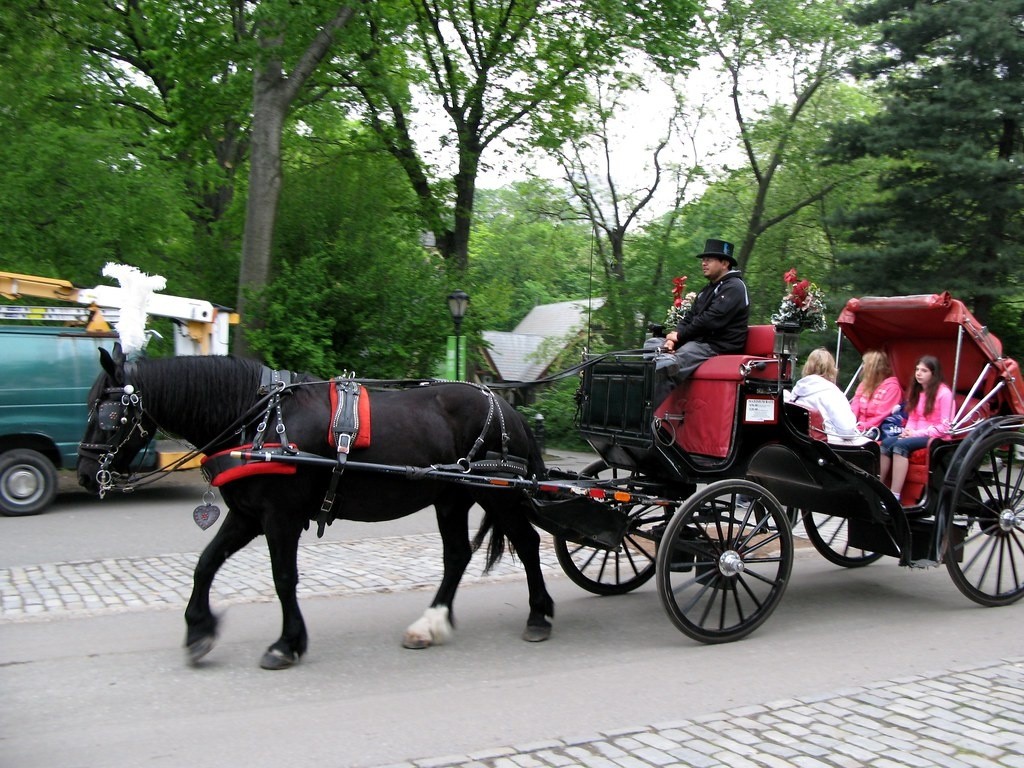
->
[635,325,774,461]
[783,400,880,479]
[894,392,989,503]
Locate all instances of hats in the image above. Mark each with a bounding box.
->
[696,238,738,267]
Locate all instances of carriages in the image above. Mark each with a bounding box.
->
[76,292,1024,672]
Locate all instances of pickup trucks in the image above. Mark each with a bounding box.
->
[0,323,160,517]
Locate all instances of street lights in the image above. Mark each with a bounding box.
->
[446,288,471,381]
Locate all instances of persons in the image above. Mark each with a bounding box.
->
[643,239,749,414]
[790,348,873,446]
[851,351,902,441]
[880,355,956,506]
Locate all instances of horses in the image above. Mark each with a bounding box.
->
[76,341,555,670]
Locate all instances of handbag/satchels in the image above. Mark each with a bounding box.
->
[879,411,909,438]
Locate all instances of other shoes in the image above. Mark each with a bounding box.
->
[655,359,680,381]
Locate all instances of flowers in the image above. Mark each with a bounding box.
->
[665,276,697,330]
[771,268,828,333]
